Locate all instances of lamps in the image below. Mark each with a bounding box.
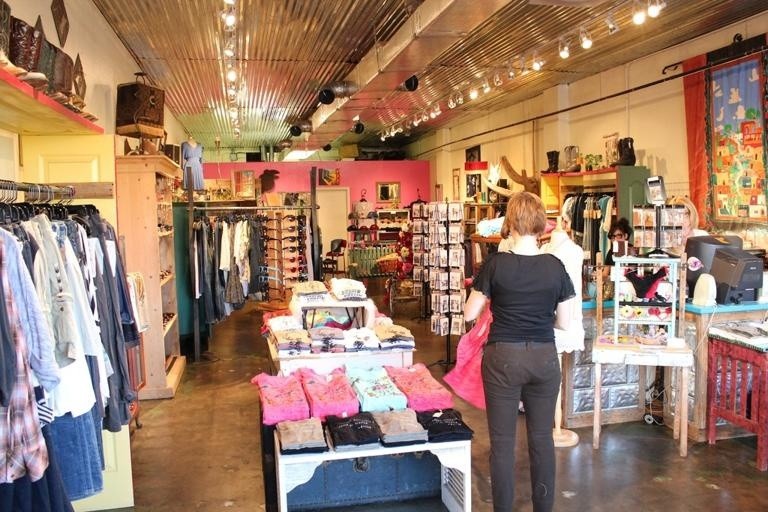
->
[220,0,242,139]
[468,51,546,100]
[635,176,680,259]
[557,9,619,61]
[632,3,661,27]
[382,88,465,142]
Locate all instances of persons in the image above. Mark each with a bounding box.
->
[538,229,585,354]
[600,217,636,277]
[352,189,376,212]
[462,189,578,511]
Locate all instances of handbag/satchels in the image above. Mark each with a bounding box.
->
[115,73,165,138]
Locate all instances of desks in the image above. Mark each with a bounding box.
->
[257,397,473,512]
[300,297,367,328]
[592,338,694,457]
[706,338,768,473]
[265,336,417,377]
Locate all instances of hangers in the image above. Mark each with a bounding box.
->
[576,185,617,204]
[0,180,100,240]
[192,207,264,229]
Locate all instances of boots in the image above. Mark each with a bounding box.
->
[610,137,636,166]
[1,0,99,123]
[541,145,581,173]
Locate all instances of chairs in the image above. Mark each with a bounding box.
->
[326,239,347,278]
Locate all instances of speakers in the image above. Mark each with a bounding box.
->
[116,83,165,138]
[487,179,507,203]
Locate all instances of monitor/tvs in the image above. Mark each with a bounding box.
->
[684,236,763,304]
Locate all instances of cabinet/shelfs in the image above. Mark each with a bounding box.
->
[115,153,188,401]
[539,165,651,256]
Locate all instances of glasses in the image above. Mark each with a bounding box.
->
[258,215,308,304]
[611,234,625,239]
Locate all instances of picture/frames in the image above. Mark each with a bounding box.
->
[231,168,255,200]
[376,182,401,203]
[466,145,480,197]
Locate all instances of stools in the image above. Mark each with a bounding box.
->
[322,258,337,281]
